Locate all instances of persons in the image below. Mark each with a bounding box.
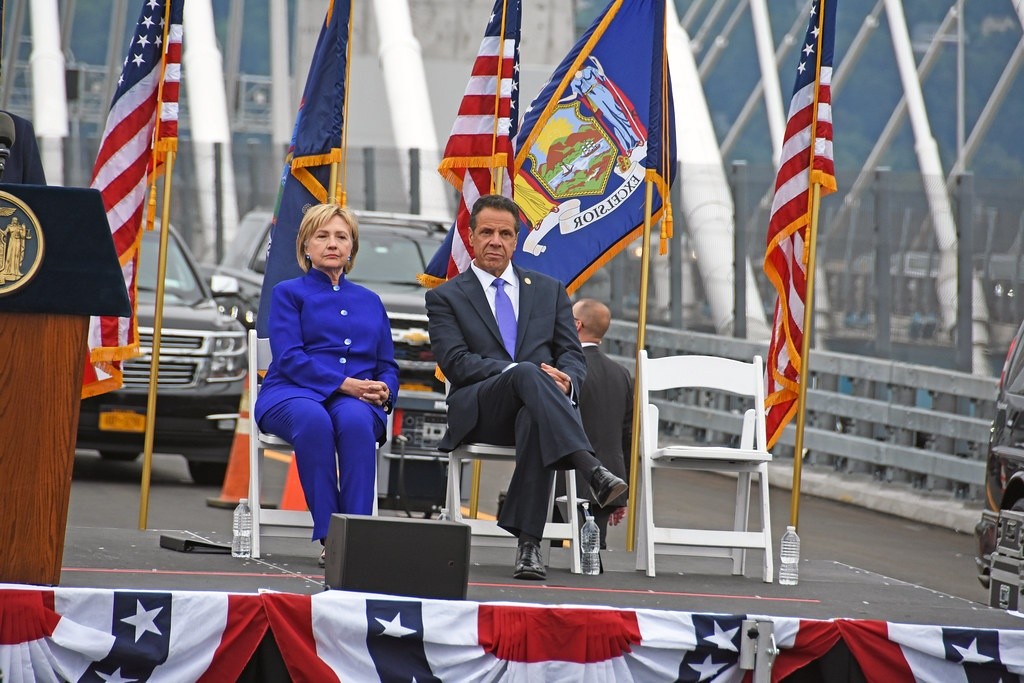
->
[425,195,627,580]
[254,203,400,566]
[0,111,47,185]
[549,298,634,550]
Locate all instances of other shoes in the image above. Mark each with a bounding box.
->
[318,546,326,567]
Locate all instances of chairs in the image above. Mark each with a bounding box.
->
[248,329,382,559]
[446,375,582,575]
[638,349,774,585]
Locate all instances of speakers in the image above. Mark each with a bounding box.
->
[324,513,471,603]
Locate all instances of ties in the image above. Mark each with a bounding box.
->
[492,278,517,361]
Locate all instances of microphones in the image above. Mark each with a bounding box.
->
[0,112,15,182]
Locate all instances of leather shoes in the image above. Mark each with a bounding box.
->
[588,465,628,509]
[513,542,546,579]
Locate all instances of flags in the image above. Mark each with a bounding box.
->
[762,0,837,450]
[416,0,677,396]
[255,0,352,339]
[80,0,182,400]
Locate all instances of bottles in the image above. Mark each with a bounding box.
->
[779,525,801,586]
[231,497,252,558]
[581,516,601,575]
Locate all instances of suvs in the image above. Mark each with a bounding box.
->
[206,210,453,396]
[972,317,1024,590]
[75,210,250,488]
